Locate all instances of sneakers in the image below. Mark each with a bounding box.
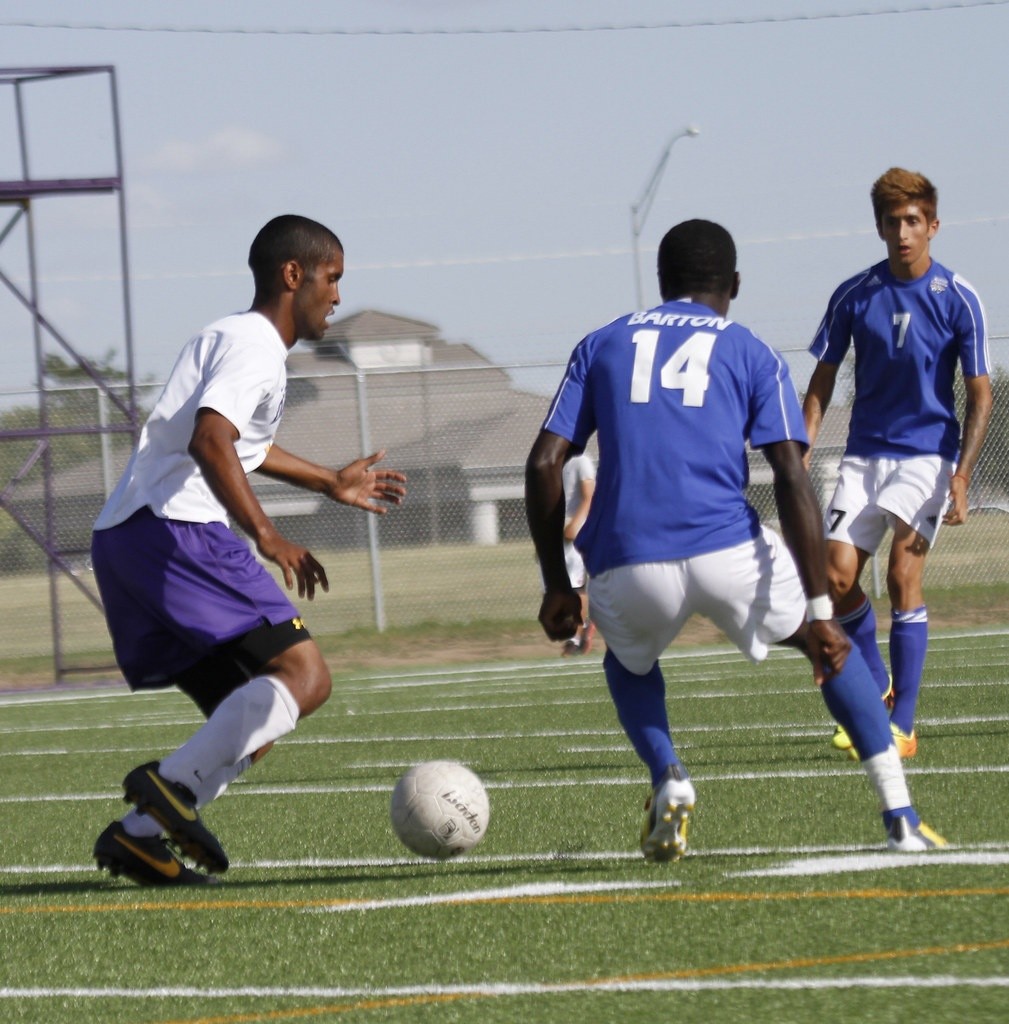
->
[888,816,954,853]
[642,767,698,865]
[831,674,918,760]
[92,820,207,886]
[122,760,230,873]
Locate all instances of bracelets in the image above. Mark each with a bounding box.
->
[952,472,968,486]
[806,594,833,623]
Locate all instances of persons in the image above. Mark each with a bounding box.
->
[800,169,992,761]
[534,455,597,656]
[91,216,409,887]
[525,218,949,862]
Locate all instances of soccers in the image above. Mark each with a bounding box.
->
[390,759,493,860]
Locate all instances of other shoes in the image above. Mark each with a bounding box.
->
[562,640,596,657]
